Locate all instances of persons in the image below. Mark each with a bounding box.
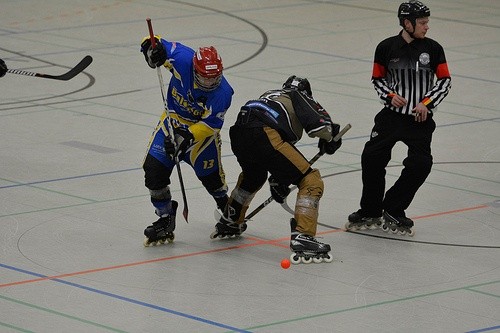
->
[143,35,235,247]
[344,0,452,236]
[210,75,342,264]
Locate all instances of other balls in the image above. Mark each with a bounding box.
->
[281,260,290,268]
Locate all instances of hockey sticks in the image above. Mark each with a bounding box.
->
[214,123,352,224]
[6,55,93,82]
[146,18,190,224]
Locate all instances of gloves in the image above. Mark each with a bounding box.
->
[318,123,343,156]
[140,36,167,68]
[164,127,195,162]
[268,174,291,203]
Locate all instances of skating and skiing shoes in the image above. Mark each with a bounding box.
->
[289,217,332,265]
[143,200,178,247]
[210,216,248,240]
[382,199,415,236]
[345,198,383,230]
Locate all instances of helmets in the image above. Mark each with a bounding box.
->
[193,45,224,92]
[282,74,312,96]
[397,0,431,18]
